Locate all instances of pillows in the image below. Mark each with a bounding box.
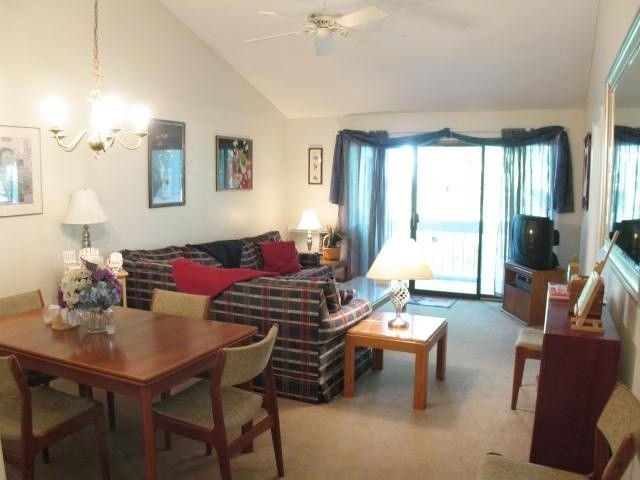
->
[261,241,301,275]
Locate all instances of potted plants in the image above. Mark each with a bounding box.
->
[322,225,345,259]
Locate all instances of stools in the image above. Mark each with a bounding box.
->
[511,329,543,410]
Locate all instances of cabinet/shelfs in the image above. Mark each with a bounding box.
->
[502,262,564,326]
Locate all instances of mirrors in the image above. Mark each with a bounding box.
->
[600,6,640,308]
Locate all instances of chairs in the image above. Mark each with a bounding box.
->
[0,288,75,463]
[0,354,107,480]
[478,378,640,480]
[106,288,211,449]
[149,322,284,480]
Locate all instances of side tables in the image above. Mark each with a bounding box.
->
[319,256,352,283]
[344,312,448,411]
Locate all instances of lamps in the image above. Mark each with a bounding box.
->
[62,189,110,248]
[367,237,434,328]
[297,211,322,252]
[42,0,149,158]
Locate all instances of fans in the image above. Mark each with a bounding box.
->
[242,0,394,50]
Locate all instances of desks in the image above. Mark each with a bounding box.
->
[528,281,622,475]
[0,306,258,480]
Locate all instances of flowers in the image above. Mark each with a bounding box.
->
[59,259,123,312]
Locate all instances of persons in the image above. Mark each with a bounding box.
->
[0,148,30,204]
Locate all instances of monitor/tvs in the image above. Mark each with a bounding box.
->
[507,214,560,271]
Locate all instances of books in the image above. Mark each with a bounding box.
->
[548,282,607,305]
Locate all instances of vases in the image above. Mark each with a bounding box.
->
[79,305,114,334]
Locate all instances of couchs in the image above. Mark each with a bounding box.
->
[120,231,372,404]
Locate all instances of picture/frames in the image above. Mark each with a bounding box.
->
[581,133,591,212]
[308,148,322,184]
[216,135,253,191]
[149,119,186,208]
[0,125,44,217]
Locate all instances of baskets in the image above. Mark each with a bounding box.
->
[322,233,342,261]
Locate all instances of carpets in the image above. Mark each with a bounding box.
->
[406,294,457,308]
